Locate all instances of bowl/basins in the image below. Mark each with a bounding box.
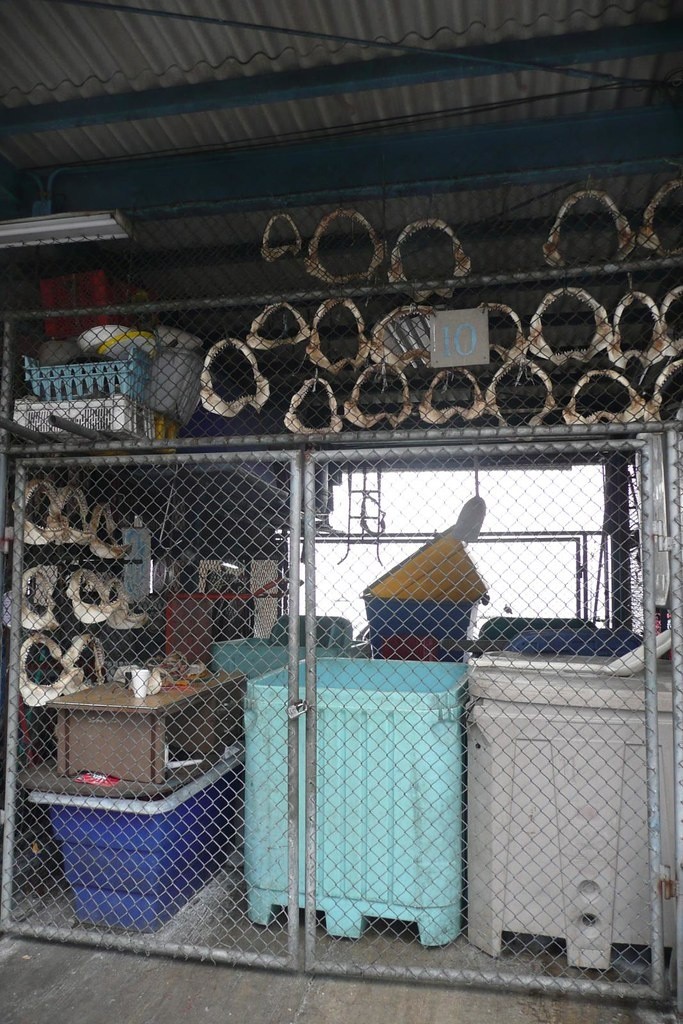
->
[76,324,133,356]
[98,331,157,360]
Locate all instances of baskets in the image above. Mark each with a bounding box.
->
[13,393,178,454]
[22,347,151,404]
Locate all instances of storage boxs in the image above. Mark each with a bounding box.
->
[28,741,244,933]
[12,349,202,456]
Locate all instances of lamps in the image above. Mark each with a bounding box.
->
[454,455,485,541]
[0,208,133,248]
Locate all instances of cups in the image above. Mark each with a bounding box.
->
[131,669,149,698]
